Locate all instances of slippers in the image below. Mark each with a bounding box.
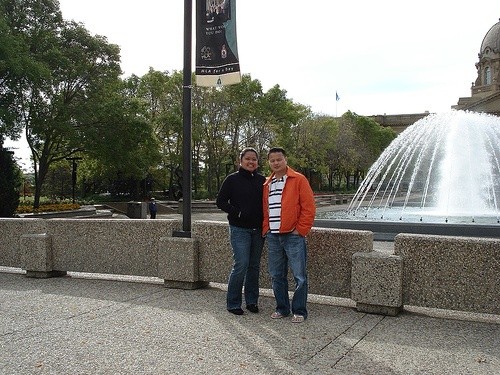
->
[291,314,304,322]
[271,311,283,319]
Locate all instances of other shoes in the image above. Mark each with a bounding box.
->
[227,308,244,315]
[246,304,259,313]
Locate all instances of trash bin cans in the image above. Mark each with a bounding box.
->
[126,201,147,219]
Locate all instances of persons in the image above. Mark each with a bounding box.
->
[149,198,157,219]
[263,148,316,322]
[216,147,266,315]
